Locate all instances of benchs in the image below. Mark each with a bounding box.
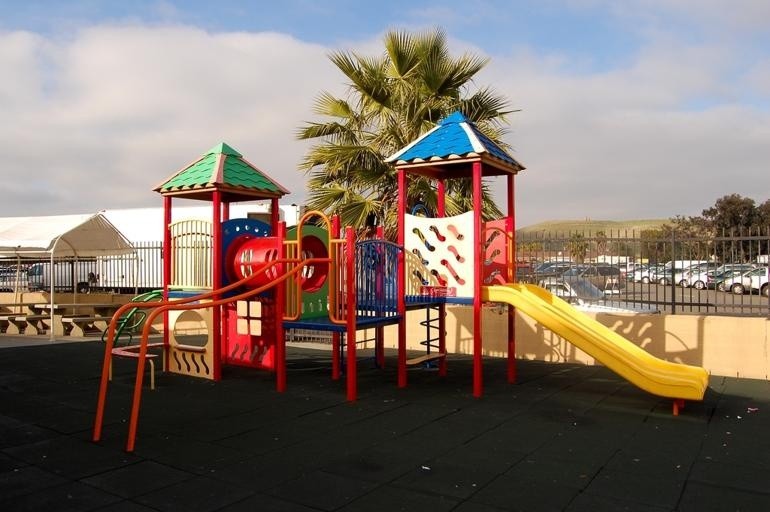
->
[0,312,132,337]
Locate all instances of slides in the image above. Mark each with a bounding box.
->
[481,283,709,401]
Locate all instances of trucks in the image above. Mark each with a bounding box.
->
[26,262,94,293]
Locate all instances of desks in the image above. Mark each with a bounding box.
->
[33,300,122,338]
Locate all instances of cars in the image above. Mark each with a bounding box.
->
[517,261,660,315]
[652,259,769,298]
